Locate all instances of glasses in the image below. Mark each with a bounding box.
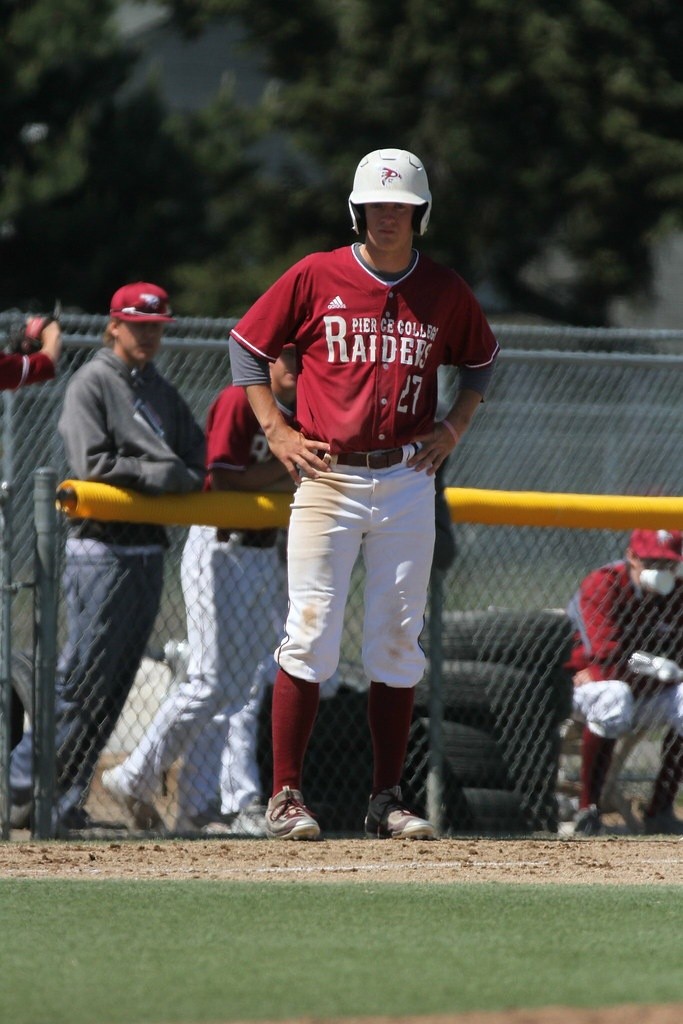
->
[109,304,172,316]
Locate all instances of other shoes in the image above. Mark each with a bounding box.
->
[576,804,606,838]
[2,790,97,838]
[101,768,168,835]
[231,807,268,834]
[174,803,231,835]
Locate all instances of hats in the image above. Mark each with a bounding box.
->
[112,282,177,322]
[631,526,683,562]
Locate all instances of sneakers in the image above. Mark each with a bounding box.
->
[364,786,437,840]
[263,785,320,841]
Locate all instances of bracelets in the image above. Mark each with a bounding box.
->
[442,419,458,444]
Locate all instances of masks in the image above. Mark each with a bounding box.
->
[636,563,675,596]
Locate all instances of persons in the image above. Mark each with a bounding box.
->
[0,315,64,752]
[228,149,502,842]
[566,648,593,686]
[100,344,297,836]
[572,526,683,836]
[11,281,208,823]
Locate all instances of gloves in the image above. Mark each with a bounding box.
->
[628,651,683,684]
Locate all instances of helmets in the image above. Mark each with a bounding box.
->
[347,148,432,236]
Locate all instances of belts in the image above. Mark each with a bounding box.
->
[218,525,277,550]
[316,442,420,471]
